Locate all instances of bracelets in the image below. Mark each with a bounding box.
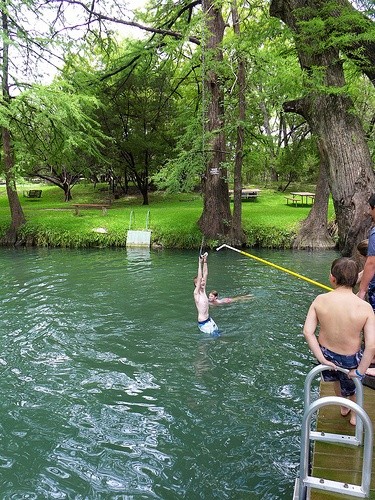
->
[203,261,207,263]
[355,370,366,380]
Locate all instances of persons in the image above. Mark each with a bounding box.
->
[303,257,375,425]
[357,193,375,376]
[193,252,218,334]
[208,290,221,305]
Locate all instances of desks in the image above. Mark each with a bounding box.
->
[291,192,315,205]
[229,188,259,194]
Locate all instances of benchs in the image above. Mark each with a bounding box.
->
[72,202,110,215]
[284,196,300,207]
[22,190,43,198]
[242,195,256,199]
